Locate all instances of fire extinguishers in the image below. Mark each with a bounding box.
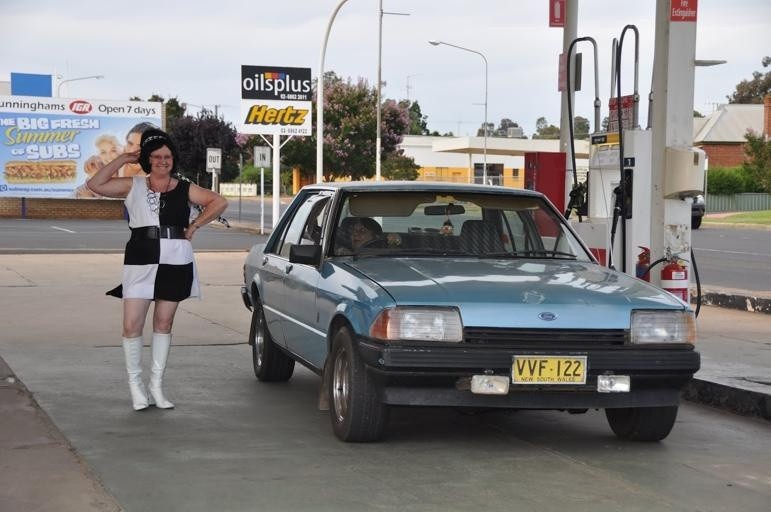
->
[636,245,650,283]
[645,247,689,304]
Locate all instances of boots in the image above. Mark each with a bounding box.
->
[148,331,177,409]
[120,334,151,411]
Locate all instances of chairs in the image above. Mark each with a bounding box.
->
[333,217,509,259]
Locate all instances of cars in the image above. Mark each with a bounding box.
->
[692,194,706,230]
[240,180,701,443]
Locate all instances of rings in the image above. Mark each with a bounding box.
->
[397,238,400,241]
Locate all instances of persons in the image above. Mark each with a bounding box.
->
[334,216,402,258]
[87,127,229,412]
[75,134,131,197]
[84,122,160,177]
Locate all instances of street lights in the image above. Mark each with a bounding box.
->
[56,75,105,98]
[429,40,489,184]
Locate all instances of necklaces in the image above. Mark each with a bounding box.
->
[148,175,171,192]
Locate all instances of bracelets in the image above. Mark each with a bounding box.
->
[191,219,200,230]
